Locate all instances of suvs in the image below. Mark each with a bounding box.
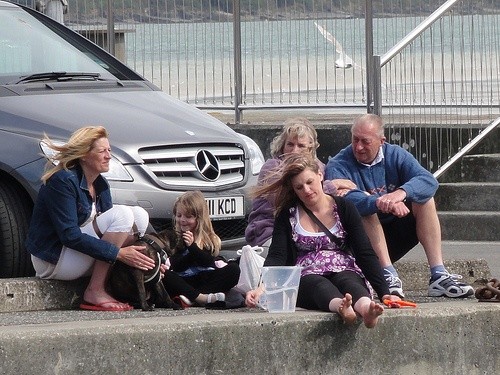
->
[0,1,268,278]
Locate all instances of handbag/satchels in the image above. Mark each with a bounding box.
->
[231,245,266,295]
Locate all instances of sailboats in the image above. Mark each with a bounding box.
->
[312,20,353,69]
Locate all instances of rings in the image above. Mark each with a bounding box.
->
[138,262,142,266]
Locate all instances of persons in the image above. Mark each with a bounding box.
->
[244,118,358,257]
[243,152,402,330]
[157,190,240,312]
[325,111,476,300]
[30,124,157,310]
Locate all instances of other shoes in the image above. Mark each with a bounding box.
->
[180,295,194,306]
[207,293,225,304]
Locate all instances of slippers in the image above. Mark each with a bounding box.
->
[79,301,133,310]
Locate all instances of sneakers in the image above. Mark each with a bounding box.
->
[428,270,473,299]
[384,268,404,298]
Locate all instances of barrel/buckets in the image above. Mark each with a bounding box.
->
[261,266,301,313]
